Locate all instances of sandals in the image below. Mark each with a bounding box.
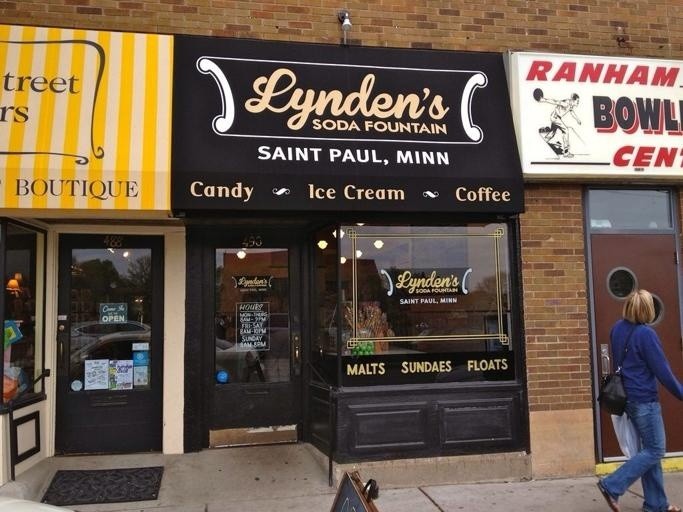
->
[597,479,619,511]
[667,504,680,511]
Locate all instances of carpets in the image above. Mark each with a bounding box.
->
[39,465,164,506]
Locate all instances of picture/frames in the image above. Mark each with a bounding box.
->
[411,224,469,296]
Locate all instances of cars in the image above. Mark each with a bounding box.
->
[22,321,267,392]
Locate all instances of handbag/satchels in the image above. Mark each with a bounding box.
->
[597,372,628,417]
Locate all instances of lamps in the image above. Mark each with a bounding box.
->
[6,279,24,298]
[14,273,23,282]
[338,10,353,45]
[131,295,145,305]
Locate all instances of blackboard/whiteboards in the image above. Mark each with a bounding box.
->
[330,471,373,512]
[235,302,270,353]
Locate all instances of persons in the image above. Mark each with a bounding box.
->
[597,289,683,512]
[215,315,229,340]
